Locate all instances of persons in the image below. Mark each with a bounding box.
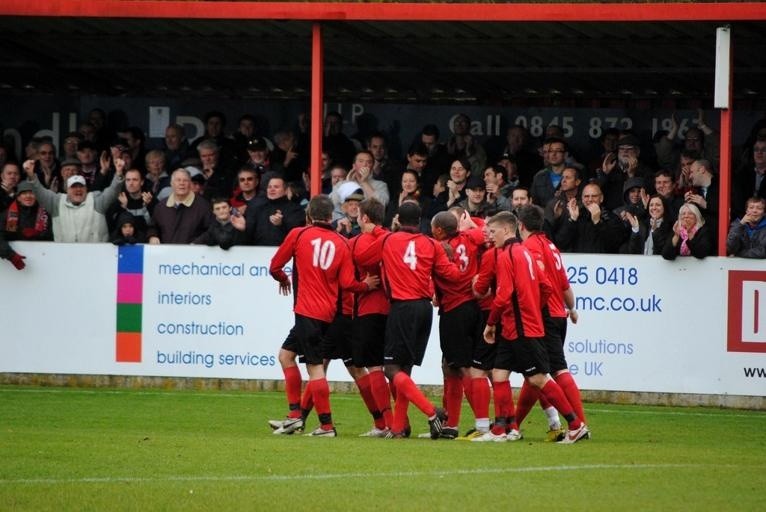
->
[1,107,766,259]
[267,195,592,444]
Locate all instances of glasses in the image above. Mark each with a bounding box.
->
[4,117,765,201]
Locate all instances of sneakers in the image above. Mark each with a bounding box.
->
[555,421,592,444]
[415,425,459,440]
[267,418,305,435]
[427,406,449,441]
[452,427,523,442]
[358,426,390,437]
[302,426,338,437]
[385,426,411,439]
[543,424,566,442]
